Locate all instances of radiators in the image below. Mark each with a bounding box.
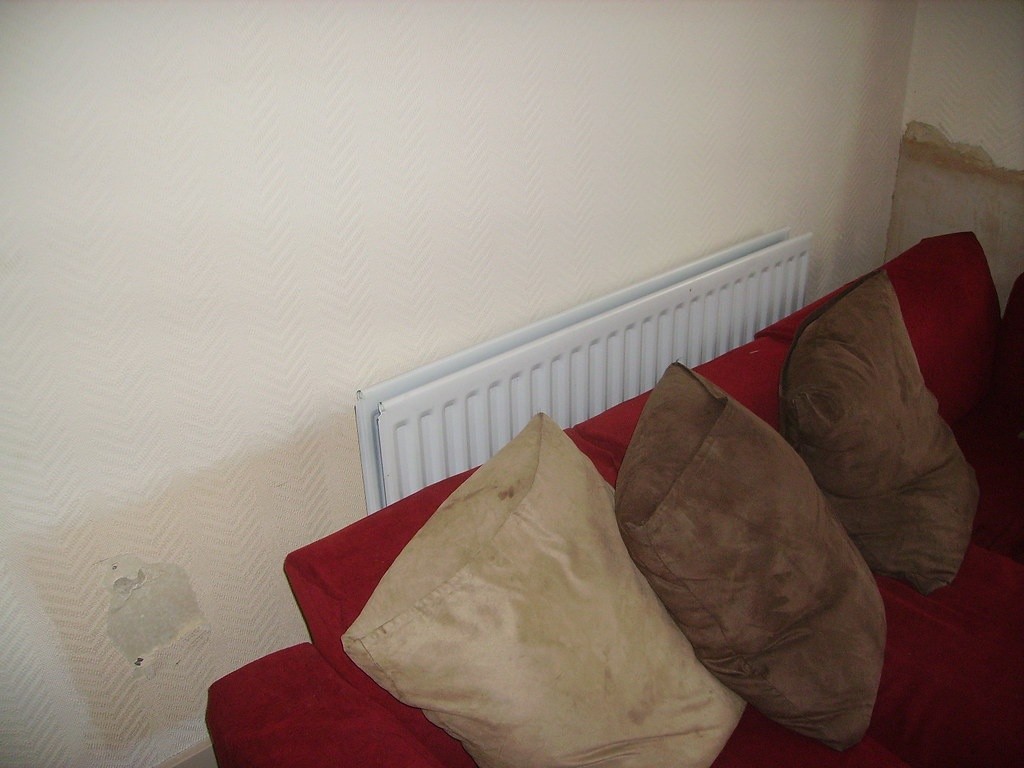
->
[354,227,809,513]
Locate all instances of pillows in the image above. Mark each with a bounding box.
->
[625,364,887,752]
[341,413,747,768]
[782,268,976,592]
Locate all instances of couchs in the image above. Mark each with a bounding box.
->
[203,232,1024,768]
